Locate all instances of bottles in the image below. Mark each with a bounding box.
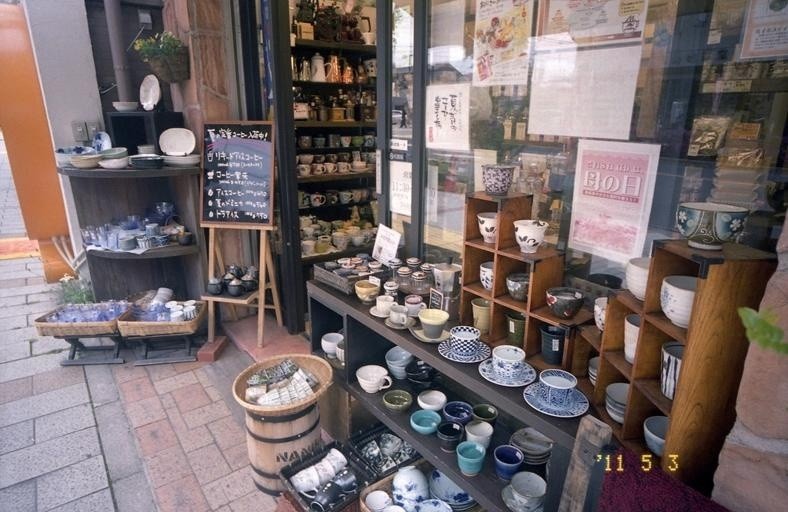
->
[308,88,376,121]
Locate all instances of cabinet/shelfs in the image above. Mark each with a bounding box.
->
[55,165,210,303]
[306,278,613,511]
[462,190,594,374]
[569,237,776,484]
[288,32,378,263]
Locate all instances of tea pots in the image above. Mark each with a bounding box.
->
[206,264,259,297]
[291,52,369,85]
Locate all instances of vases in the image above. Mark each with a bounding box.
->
[146,51,190,82]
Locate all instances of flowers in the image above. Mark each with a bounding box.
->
[132,29,185,58]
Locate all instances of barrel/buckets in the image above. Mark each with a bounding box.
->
[244,401,322,496]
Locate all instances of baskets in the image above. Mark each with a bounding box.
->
[232,354,335,417]
[359,457,487,512]
[33,300,133,336]
[117,290,208,337]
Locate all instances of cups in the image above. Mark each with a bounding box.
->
[164,300,197,322]
[510,472,547,509]
[289,432,422,511]
[326,255,577,407]
[243,358,320,408]
[436,421,465,453]
[79,214,192,250]
[363,32,376,45]
[465,421,493,450]
[494,445,524,481]
[292,132,377,255]
[457,442,486,475]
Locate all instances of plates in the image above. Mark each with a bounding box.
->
[502,484,547,512]
[410,410,442,433]
[510,426,554,466]
[52,127,202,168]
[365,467,478,512]
[368,305,589,417]
[111,101,139,113]
[140,74,163,112]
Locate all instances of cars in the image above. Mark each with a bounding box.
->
[392,109,407,127]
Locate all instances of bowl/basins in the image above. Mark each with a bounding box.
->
[677,201,750,251]
[391,465,430,511]
[588,258,699,456]
